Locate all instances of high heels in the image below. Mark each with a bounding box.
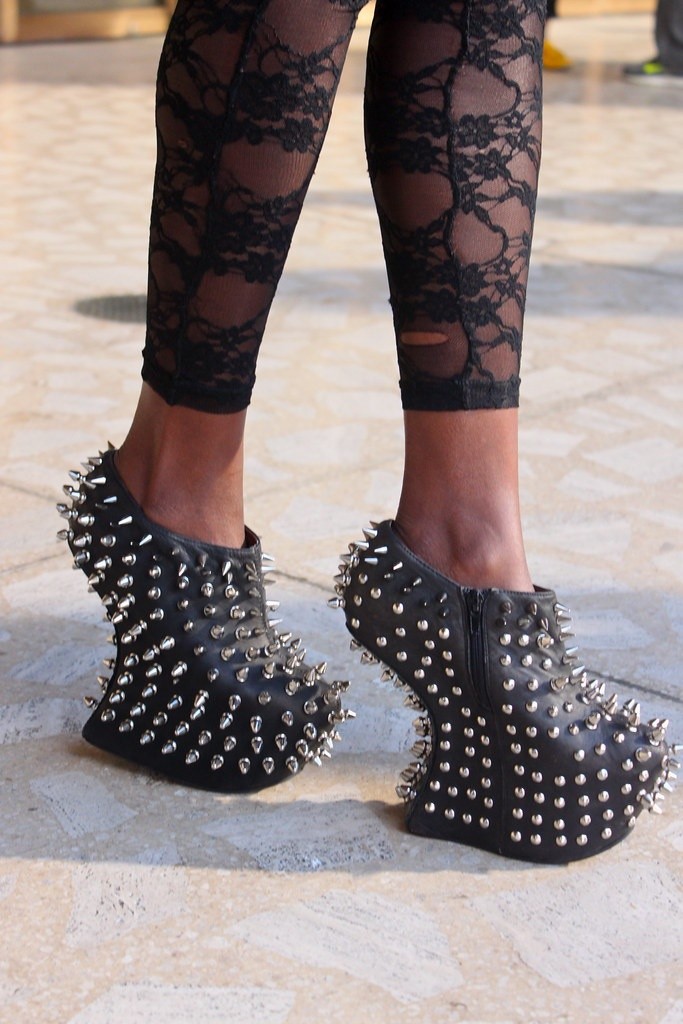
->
[58,439,356,794]
[327,518,682,865]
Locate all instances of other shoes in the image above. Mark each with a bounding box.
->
[542,39,572,70]
[623,58,683,89]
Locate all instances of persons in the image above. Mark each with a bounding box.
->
[53,0,683,863]
